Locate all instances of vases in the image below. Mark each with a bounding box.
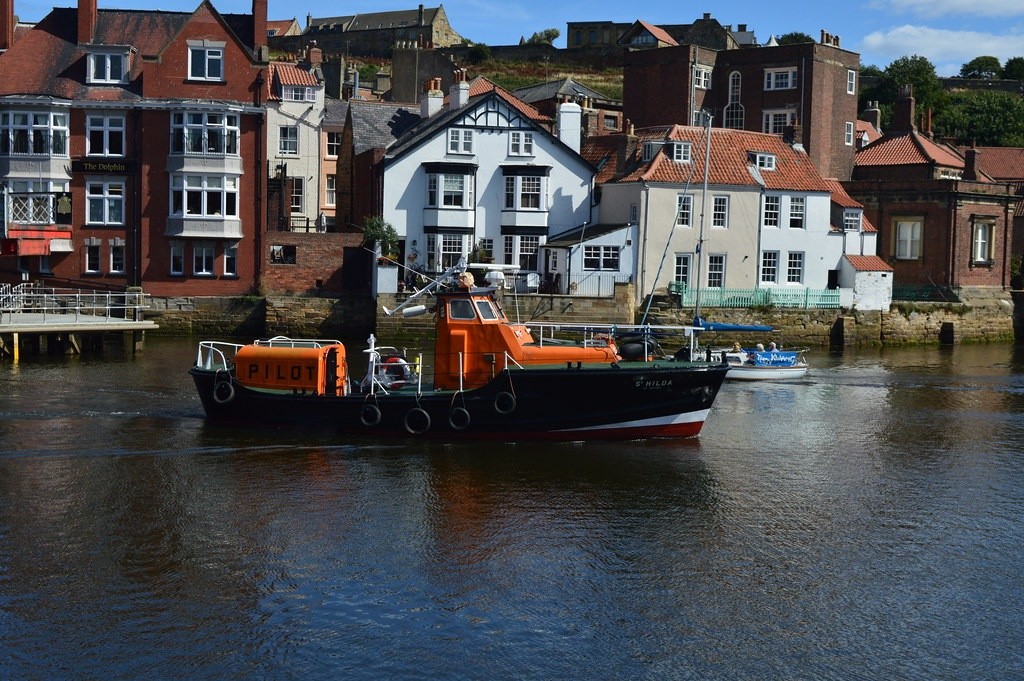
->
[388,260,396,265]
[379,262,385,265]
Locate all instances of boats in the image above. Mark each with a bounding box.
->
[187,247,733,442]
[660,315,810,381]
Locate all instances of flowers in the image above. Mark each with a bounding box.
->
[378,256,388,263]
[387,254,397,260]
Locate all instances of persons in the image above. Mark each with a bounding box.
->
[769,342,779,352]
[757,343,765,352]
[729,342,741,353]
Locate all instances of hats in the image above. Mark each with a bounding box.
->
[756,343,764,350]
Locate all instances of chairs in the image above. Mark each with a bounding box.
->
[548,273,560,293]
[526,273,539,294]
[540,273,553,293]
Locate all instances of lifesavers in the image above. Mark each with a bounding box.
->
[402,407,433,436]
[359,405,383,426]
[446,406,471,430]
[381,358,411,391]
[491,391,518,415]
[212,382,235,404]
